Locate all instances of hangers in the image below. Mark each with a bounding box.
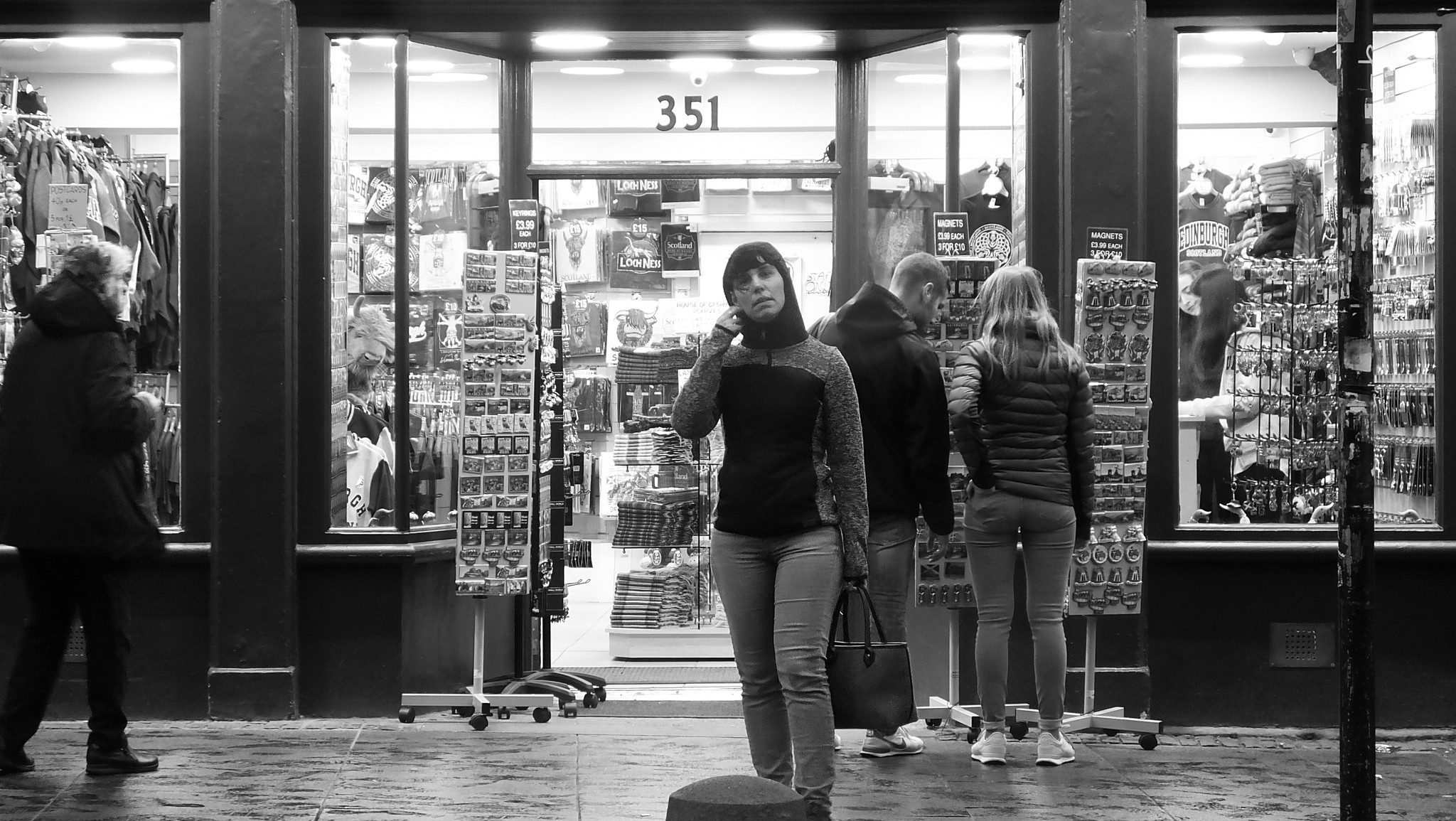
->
[165,188,173,208]
[370,374,459,435]
[25,114,158,186]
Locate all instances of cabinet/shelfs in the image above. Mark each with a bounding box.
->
[611,381,719,628]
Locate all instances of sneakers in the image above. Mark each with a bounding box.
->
[834,734,842,749]
[860,726,923,757]
[1036,731,1076,767]
[971,729,1007,765]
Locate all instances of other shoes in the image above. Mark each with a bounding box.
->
[1,744,38,775]
[86,743,160,775]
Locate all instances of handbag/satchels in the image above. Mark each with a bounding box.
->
[825,585,919,730]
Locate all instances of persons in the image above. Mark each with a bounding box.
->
[669,242,873,821]
[945,265,1097,764]
[0,244,167,776]
[805,251,956,760]
[1177,259,1299,524]
